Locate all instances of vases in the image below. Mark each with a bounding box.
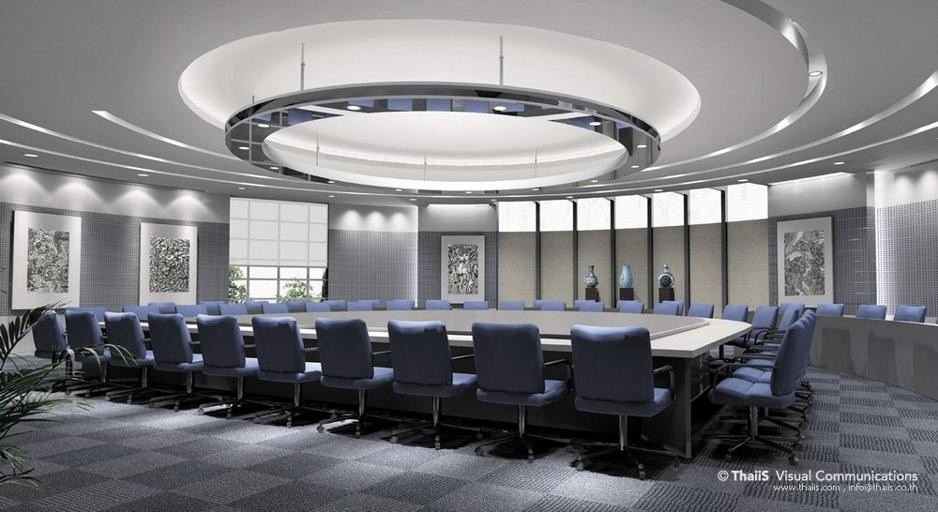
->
[657,263,676,289]
[584,264,598,287]
[619,264,633,289]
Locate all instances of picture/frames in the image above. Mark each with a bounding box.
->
[138,222,199,307]
[441,233,486,306]
[10,211,81,311]
[776,215,835,310]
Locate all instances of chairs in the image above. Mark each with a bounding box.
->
[857,305,886,320]
[893,304,926,322]
[815,304,844,315]
